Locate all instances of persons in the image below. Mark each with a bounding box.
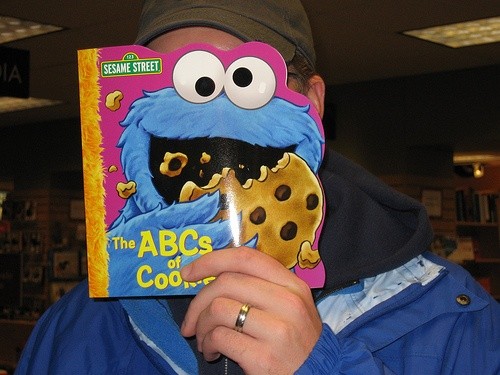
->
[14,0,500,375]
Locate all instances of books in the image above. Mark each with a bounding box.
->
[429,190,499,262]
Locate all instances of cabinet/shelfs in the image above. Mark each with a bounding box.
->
[457,221,500,300]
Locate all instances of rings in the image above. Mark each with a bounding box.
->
[235,304,250,333]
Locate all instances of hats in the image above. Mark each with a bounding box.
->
[132,0,316,80]
